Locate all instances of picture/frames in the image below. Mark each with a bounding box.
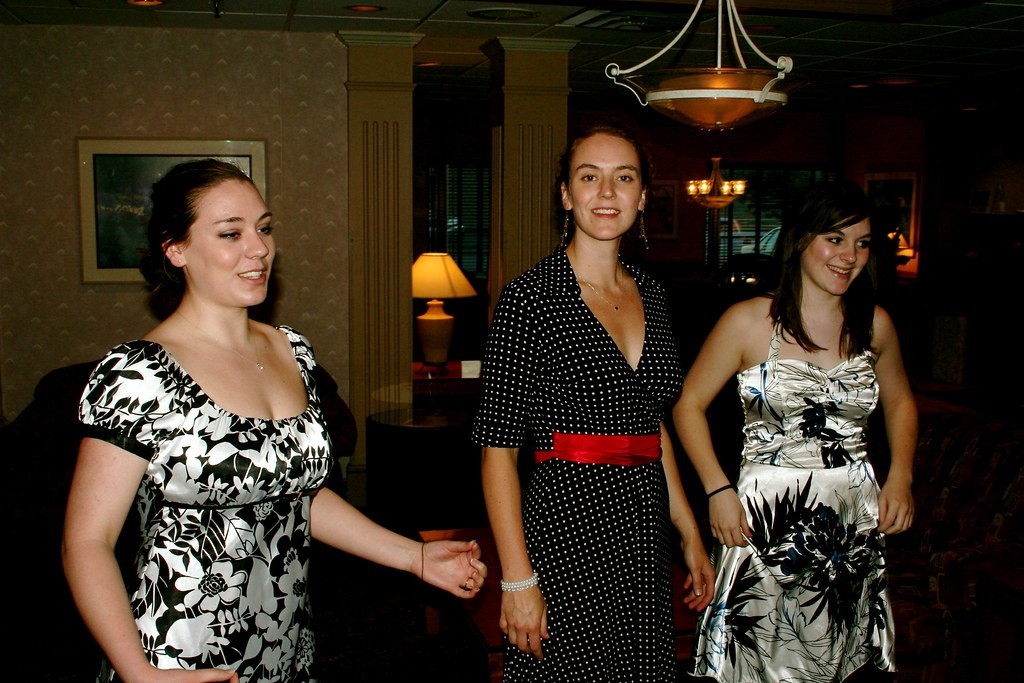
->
[73,135,269,287]
[629,180,681,240]
[864,172,924,280]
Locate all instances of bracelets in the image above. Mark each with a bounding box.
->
[421,542,425,581]
[497,572,540,592]
[707,484,735,498]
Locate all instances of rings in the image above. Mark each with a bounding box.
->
[464,577,473,590]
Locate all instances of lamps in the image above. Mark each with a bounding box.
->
[412,253,477,375]
[685,157,747,209]
[604,0,793,132]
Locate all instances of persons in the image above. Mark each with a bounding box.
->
[58,157,490,683]
[468,123,718,683]
[672,177,920,683]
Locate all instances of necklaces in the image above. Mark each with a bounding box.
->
[570,259,624,310]
[175,308,265,373]
[799,313,843,370]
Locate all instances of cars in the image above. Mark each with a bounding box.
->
[704,215,741,256]
[740,222,781,256]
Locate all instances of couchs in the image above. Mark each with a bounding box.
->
[0,363,359,683]
